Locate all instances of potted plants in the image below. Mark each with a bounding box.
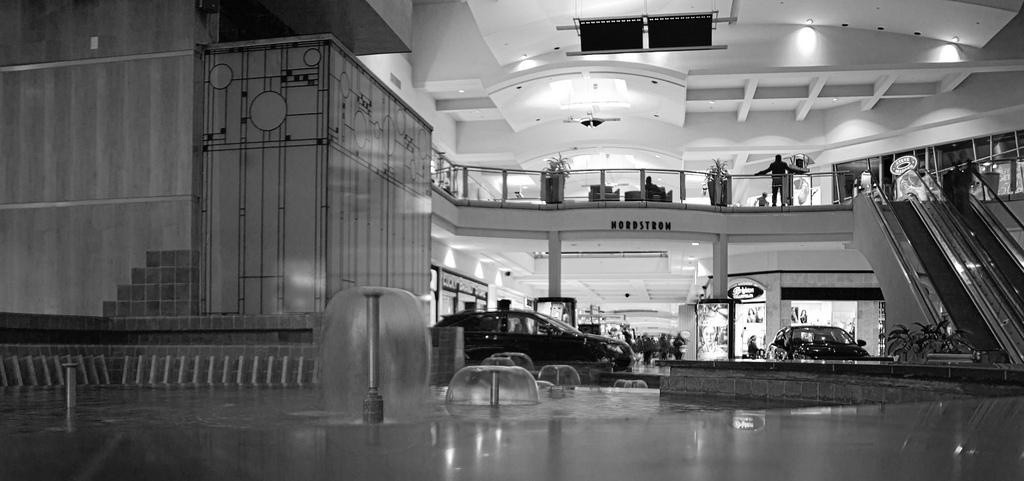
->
[544,153,573,203]
[704,158,729,206]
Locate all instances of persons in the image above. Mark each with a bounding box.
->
[645,177,657,192]
[621,330,686,367]
[698,326,727,360]
[748,336,759,359]
[755,155,803,206]
[754,193,769,207]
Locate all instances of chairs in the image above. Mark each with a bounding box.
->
[588,185,619,202]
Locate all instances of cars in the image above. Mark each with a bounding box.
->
[435,306,635,371]
[770,323,873,360]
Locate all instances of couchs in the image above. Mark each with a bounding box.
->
[624,187,673,202]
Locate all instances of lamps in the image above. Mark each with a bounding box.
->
[556,0,738,57]
